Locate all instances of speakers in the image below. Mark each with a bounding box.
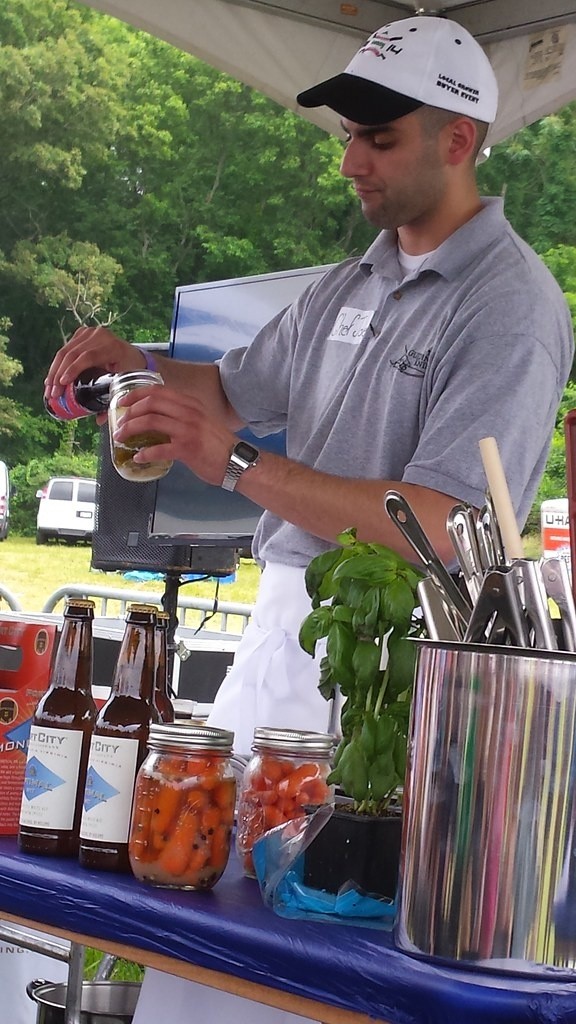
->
[90,343,240,577]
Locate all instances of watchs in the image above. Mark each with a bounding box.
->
[221,439,261,493]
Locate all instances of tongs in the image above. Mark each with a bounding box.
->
[460,554,575,654]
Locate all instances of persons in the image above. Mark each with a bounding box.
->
[41,16,576,1024]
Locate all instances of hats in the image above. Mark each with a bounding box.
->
[296,16,499,126]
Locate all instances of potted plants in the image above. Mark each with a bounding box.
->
[299,528,429,905]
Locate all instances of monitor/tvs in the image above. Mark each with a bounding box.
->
[147,263,337,546]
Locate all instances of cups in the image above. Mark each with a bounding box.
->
[392,635,576,983]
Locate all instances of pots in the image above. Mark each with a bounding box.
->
[26,978,142,1024]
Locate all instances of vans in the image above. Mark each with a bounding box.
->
[1,460,16,540]
[36,477,97,546]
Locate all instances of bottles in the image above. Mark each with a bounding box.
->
[78,606,158,874]
[151,610,176,723]
[42,369,135,422]
[19,598,99,856]
